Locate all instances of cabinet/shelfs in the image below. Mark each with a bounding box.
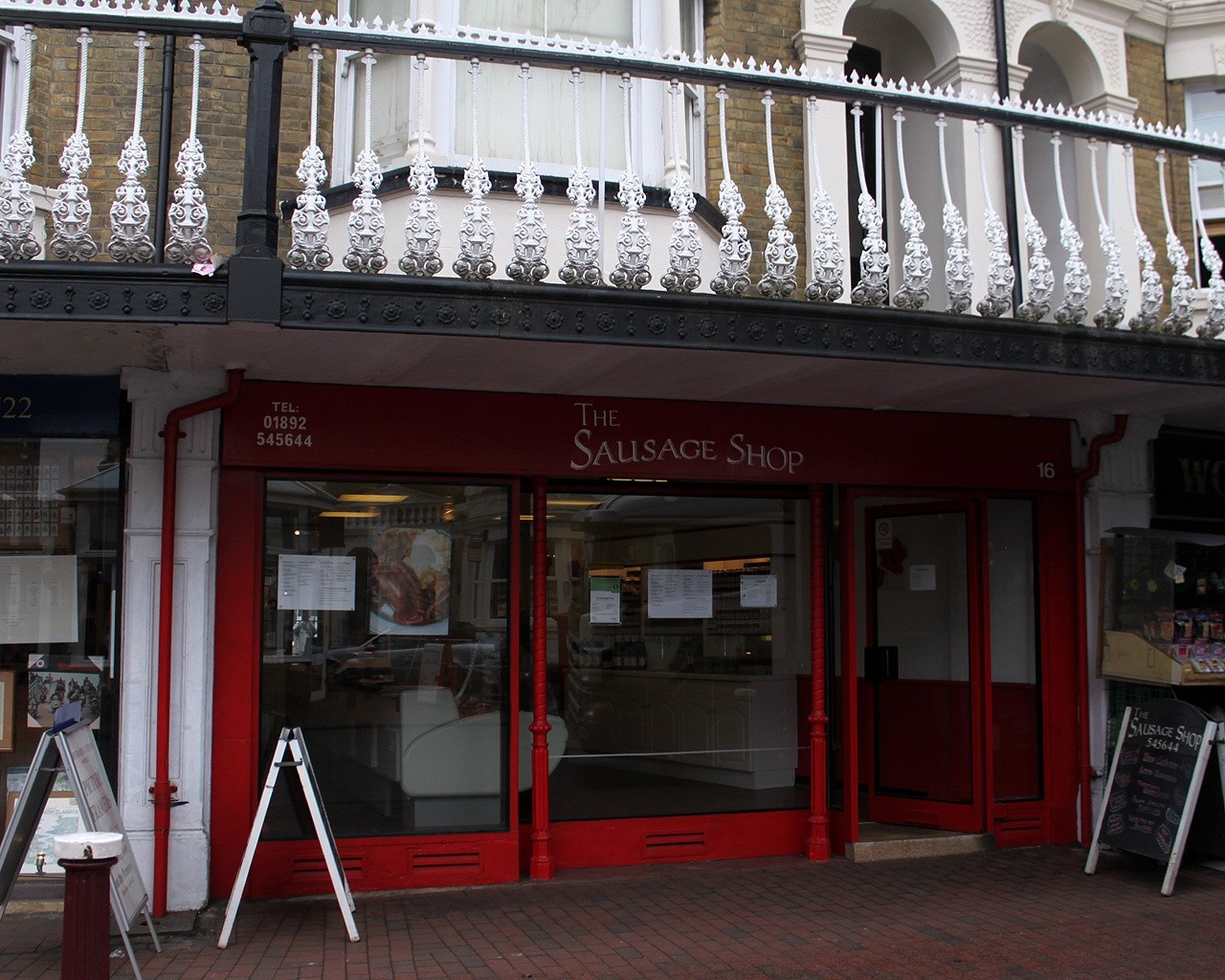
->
[561,668,800,792]
[574,510,799,675]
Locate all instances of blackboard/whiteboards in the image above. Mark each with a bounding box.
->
[1095,698,1225,863]
[216,725,362,950]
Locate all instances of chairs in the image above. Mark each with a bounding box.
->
[311,680,570,797]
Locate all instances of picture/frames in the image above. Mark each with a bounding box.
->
[0,655,106,883]
